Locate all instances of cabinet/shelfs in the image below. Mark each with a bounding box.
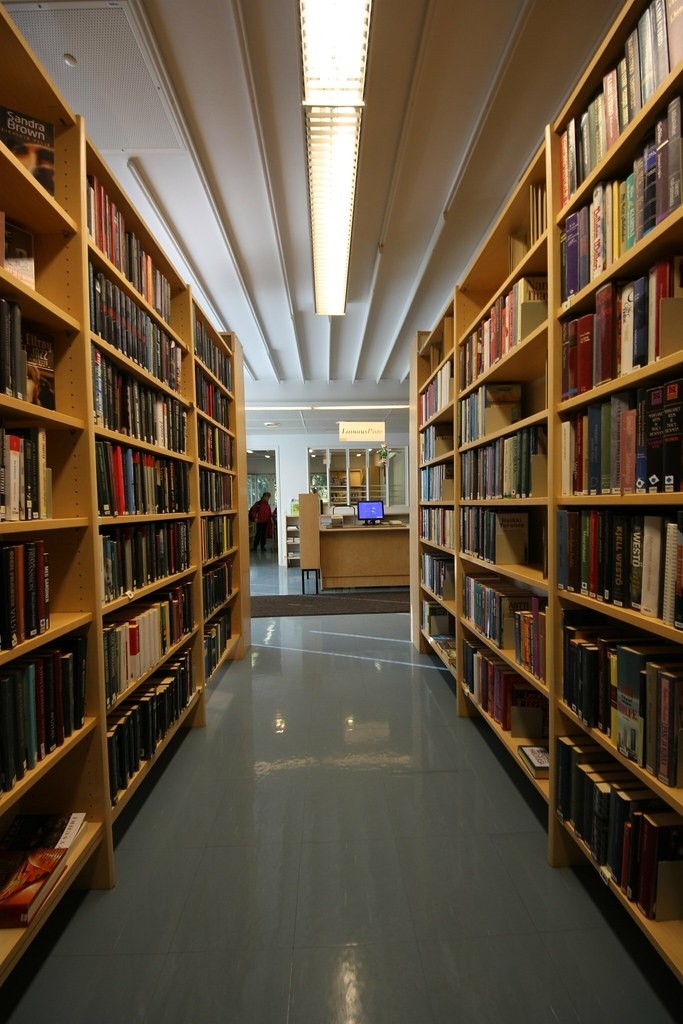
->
[285,515,300,569]
[407,0,683,982]
[0,5,250,996]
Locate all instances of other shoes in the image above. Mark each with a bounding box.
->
[253,546,257,551]
[261,546,266,551]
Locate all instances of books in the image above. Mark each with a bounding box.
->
[0,107,233,928]
[419,0,683,920]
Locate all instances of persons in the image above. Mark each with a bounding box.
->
[249,492,272,552]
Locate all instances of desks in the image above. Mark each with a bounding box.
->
[318,524,410,589]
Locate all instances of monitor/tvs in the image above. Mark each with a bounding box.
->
[358,501,384,520]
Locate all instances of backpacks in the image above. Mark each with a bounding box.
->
[249,508,256,522]
